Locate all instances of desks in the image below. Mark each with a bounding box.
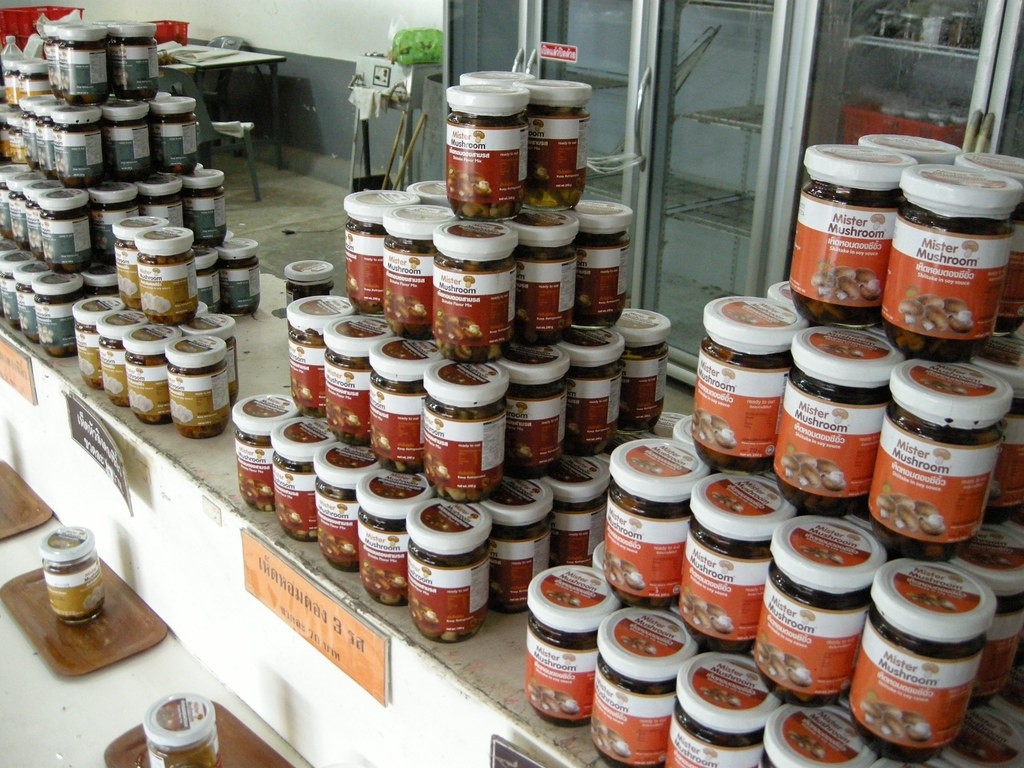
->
[161,44,286,170]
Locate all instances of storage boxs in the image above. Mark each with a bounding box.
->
[149,21,190,46]
[0,6,85,59]
[843,107,967,150]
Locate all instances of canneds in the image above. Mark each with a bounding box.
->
[0,9,1024,768]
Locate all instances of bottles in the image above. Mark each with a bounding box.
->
[388,30,443,64]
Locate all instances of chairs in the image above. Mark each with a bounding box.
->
[192,36,244,157]
[157,66,261,202]
